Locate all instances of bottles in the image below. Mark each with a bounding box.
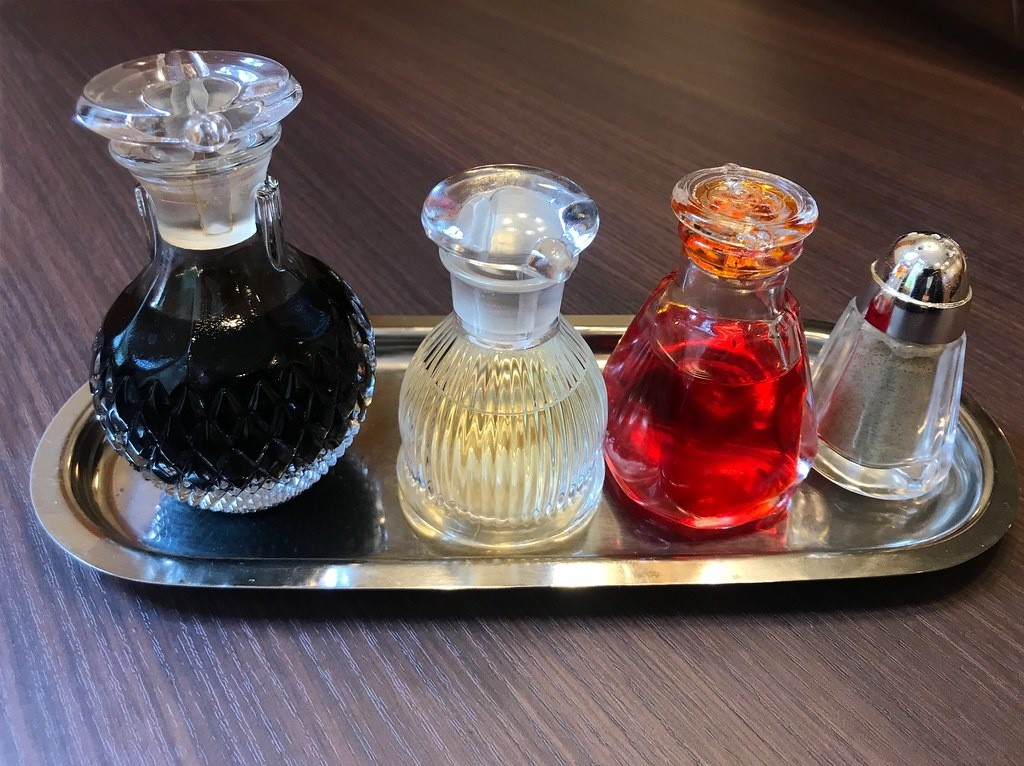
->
[76,44,378,517]
[798,229,973,504]
[398,162,607,551]
[598,162,822,532]
